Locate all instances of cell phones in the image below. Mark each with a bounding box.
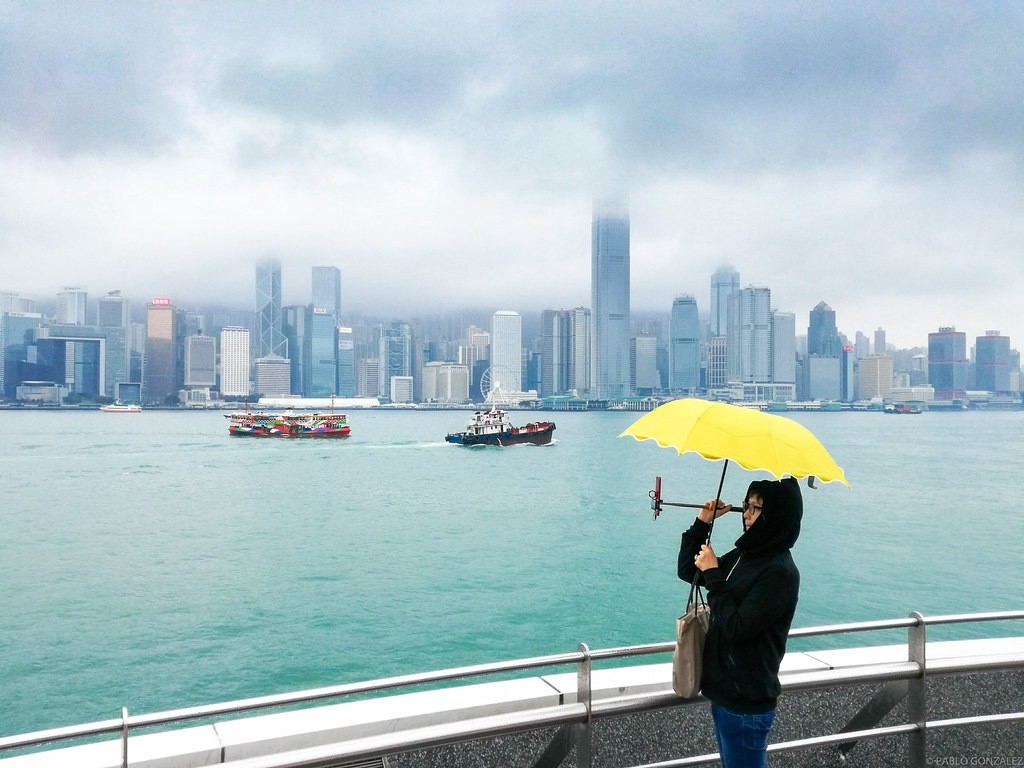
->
[655,477,662,516]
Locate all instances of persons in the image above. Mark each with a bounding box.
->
[678,479,803,768]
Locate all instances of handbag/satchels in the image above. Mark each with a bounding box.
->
[673,569,711,698]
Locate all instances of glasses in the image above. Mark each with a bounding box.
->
[741,500,763,516]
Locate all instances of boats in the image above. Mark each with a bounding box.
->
[882,400,924,414]
[446,396,556,447]
[99,402,142,412]
[229,398,352,437]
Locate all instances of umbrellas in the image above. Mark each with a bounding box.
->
[617,397,852,547]
